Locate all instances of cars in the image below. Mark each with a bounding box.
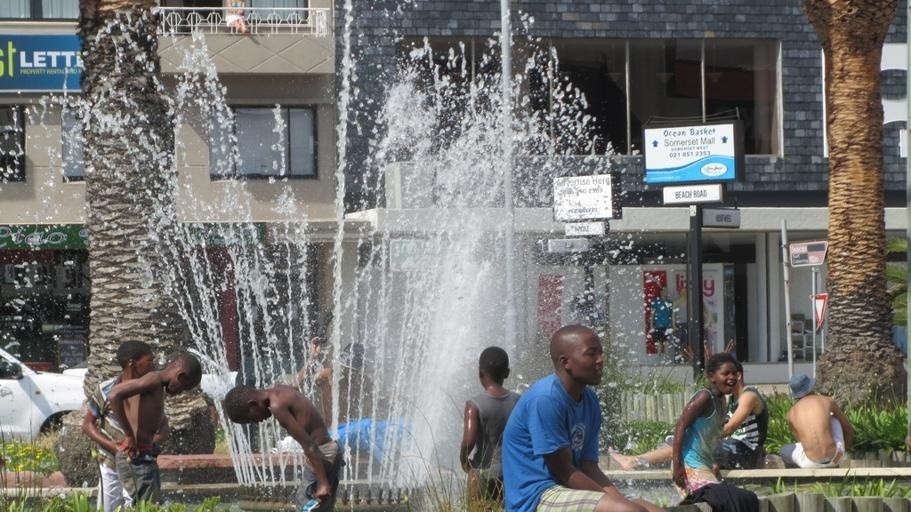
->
[0,346,239,442]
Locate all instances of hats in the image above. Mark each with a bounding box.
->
[788,372,817,399]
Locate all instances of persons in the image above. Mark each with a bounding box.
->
[671,352,759,512]
[107,353,202,511]
[780,373,853,468]
[607,360,768,470]
[501,325,669,512]
[649,286,688,361]
[224,384,342,512]
[225,0,250,34]
[289,337,392,429]
[82,340,155,512]
[459,346,523,512]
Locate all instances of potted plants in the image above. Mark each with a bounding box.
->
[747,476,908,511]
[862,388,910,462]
